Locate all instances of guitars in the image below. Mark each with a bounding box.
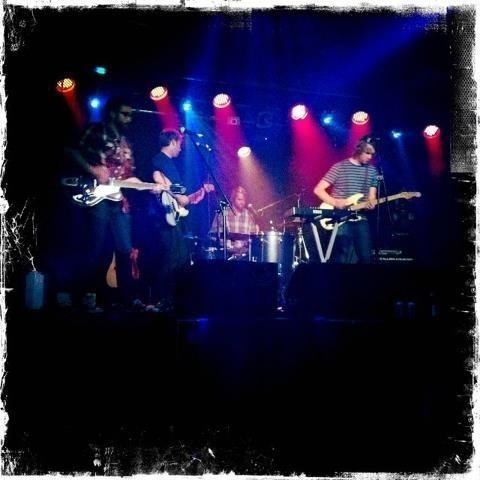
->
[318,192,422,230]
[68,169,187,209]
[106,246,140,289]
[159,183,215,228]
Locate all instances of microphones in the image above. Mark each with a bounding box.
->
[367,138,381,143]
[180,127,203,137]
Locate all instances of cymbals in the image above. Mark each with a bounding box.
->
[209,232,257,240]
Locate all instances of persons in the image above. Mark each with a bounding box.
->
[141,128,205,314]
[314,136,379,264]
[70,98,159,313]
[207,185,256,258]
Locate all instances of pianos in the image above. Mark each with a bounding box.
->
[283,207,359,223]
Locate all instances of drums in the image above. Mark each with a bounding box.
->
[199,247,230,260]
[249,230,293,278]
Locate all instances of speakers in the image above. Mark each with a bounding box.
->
[174,258,277,317]
[285,262,390,315]
